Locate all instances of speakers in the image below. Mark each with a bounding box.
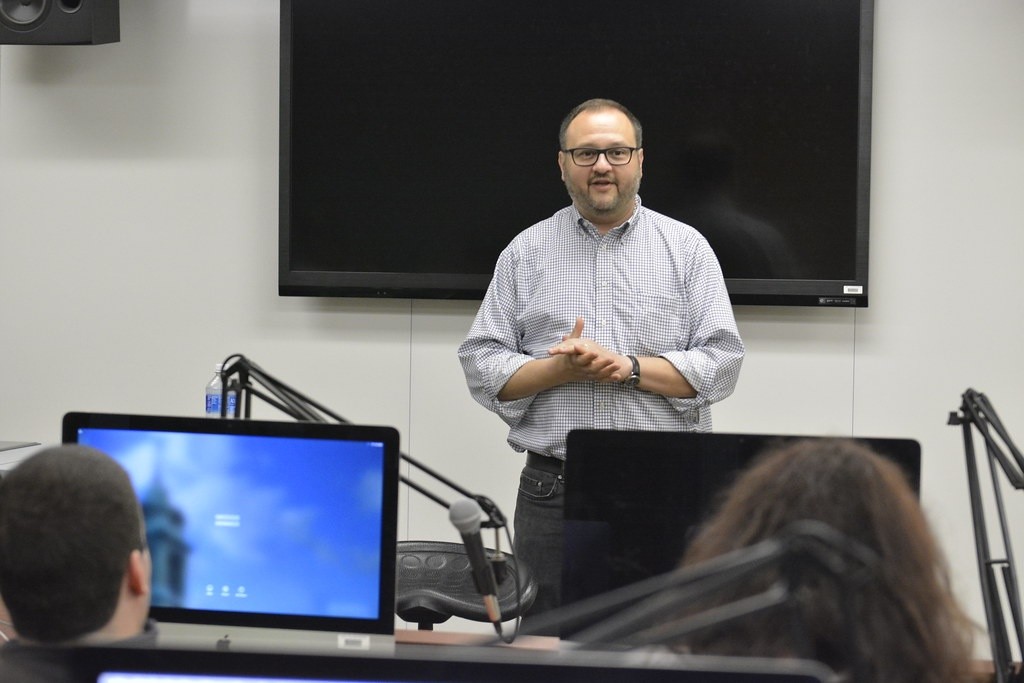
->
[0,0,121,46]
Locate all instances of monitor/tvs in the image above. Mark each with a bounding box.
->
[278,0,874,308]
[61,412,400,660]
[561,428,921,643]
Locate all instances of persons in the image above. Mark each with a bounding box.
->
[457,99,746,623]
[661,442,990,677]
[0,445,164,651]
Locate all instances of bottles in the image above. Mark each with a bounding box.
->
[205,364,236,420]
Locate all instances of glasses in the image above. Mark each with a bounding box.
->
[560,146,639,167]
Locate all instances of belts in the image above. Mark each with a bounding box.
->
[526,452,564,475]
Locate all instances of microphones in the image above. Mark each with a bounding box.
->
[449,499,504,634]
